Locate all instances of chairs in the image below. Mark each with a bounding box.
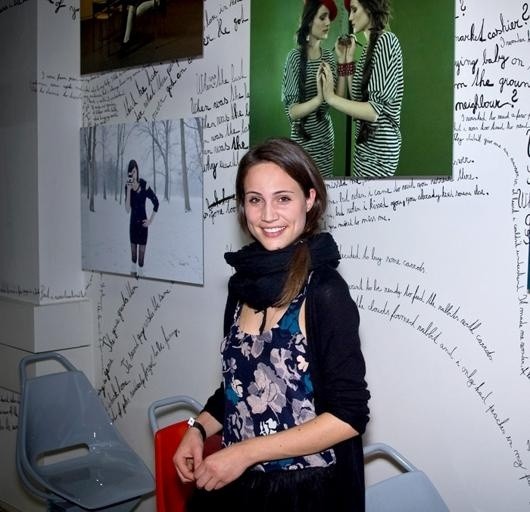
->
[149,395,226,512]
[15,353,156,511]
[363,442,451,511]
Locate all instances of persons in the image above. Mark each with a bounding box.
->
[125,159,159,267]
[172,138,370,512]
[282,0,346,177]
[322,0,404,177]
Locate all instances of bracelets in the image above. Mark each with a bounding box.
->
[186,418,207,442]
[337,62,346,76]
[346,61,355,76]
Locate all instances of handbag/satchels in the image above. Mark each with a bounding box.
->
[187,467,341,512]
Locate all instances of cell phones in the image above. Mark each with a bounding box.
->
[128,173,133,183]
